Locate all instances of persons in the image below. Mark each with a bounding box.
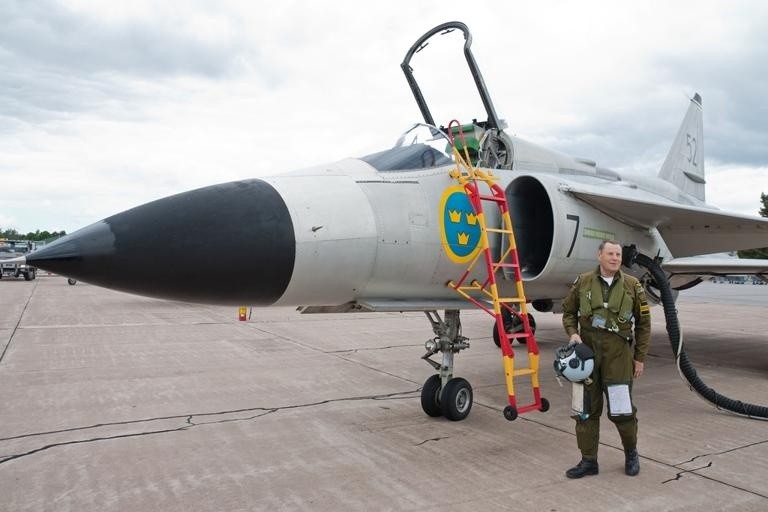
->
[562,239,651,479]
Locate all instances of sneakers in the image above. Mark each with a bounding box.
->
[624,447,640,476]
[566,460,598,478]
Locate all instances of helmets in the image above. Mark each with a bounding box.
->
[553,342,595,383]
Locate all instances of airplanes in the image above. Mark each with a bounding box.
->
[0,19,767,422]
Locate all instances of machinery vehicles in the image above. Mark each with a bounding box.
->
[0,239,37,280]
